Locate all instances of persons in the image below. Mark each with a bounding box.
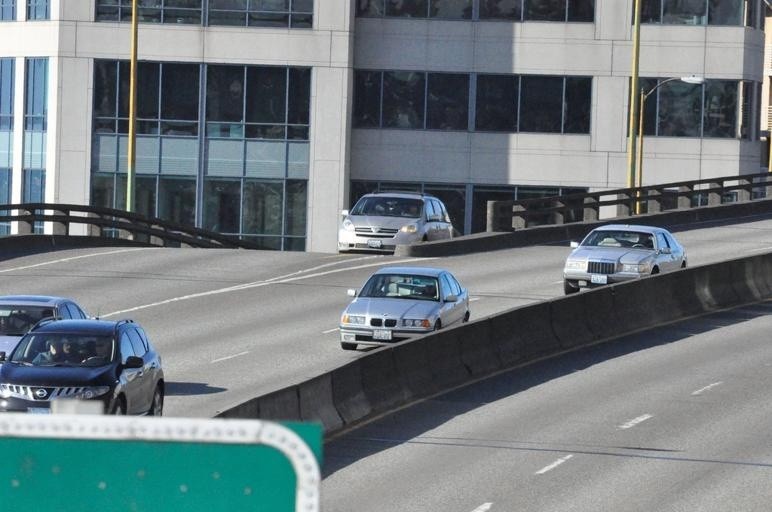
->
[60,340,80,364]
[31,339,63,366]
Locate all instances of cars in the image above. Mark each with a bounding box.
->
[340,266,471,350]
[0,294,164,415]
[564,223,688,295]
[337,192,454,254]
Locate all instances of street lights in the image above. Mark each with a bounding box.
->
[636,75,705,215]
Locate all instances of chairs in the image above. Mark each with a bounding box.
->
[378,278,438,302]
[367,199,392,218]
[599,234,653,249]
